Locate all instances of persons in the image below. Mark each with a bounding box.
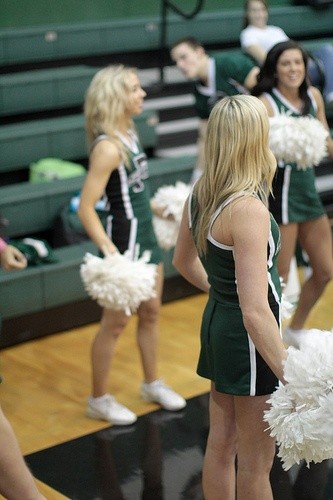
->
[249,41,333,350]
[170,0,292,117]
[77,64,187,425]
[0,239,49,500]
[172,94,289,500]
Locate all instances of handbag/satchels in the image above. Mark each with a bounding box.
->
[49,203,82,248]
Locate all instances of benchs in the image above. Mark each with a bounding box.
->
[0,0,332,320]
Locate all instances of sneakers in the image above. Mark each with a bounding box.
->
[140,380,187,412]
[87,393,137,427]
